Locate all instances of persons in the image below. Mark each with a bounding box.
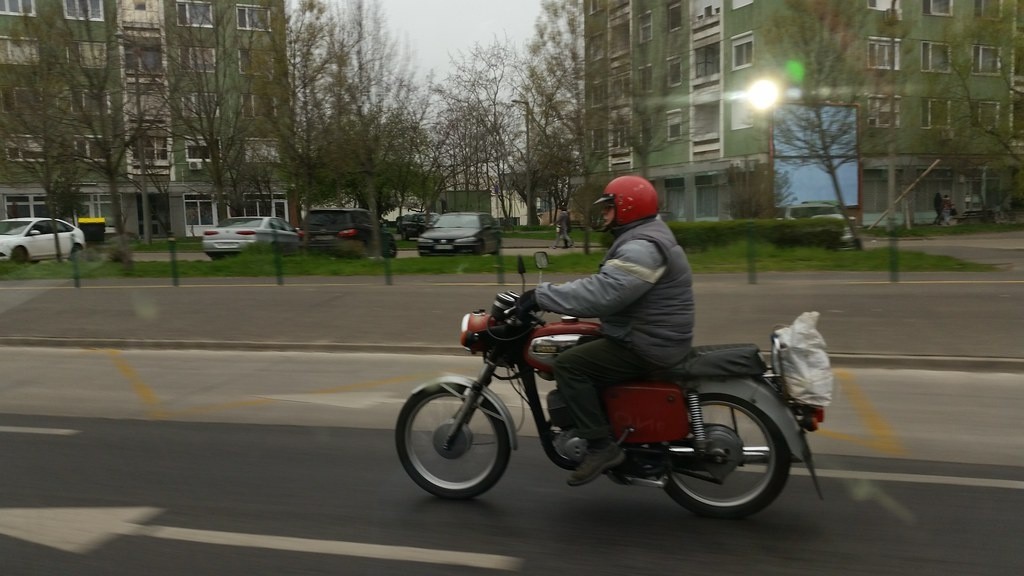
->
[930,192,953,225]
[549,205,574,250]
[516,175,696,486]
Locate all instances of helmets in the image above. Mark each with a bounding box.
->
[593,175,658,238]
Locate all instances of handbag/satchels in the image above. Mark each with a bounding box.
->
[556,224,561,234]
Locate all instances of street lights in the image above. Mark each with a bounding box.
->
[511,99,543,230]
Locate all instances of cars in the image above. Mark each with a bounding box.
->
[395,211,439,241]
[0,217,86,265]
[785,202,859,252]
[417,211,504,258]
[201,216,301,261]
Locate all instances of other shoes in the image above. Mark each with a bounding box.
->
[550,243,576,250]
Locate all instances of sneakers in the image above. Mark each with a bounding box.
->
[567,442,625,485]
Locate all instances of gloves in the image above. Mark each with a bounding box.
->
[517,289,539,326]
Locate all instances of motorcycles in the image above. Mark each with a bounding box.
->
[394,249,827,523]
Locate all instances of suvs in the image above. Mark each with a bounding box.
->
[299,207,400,259]
